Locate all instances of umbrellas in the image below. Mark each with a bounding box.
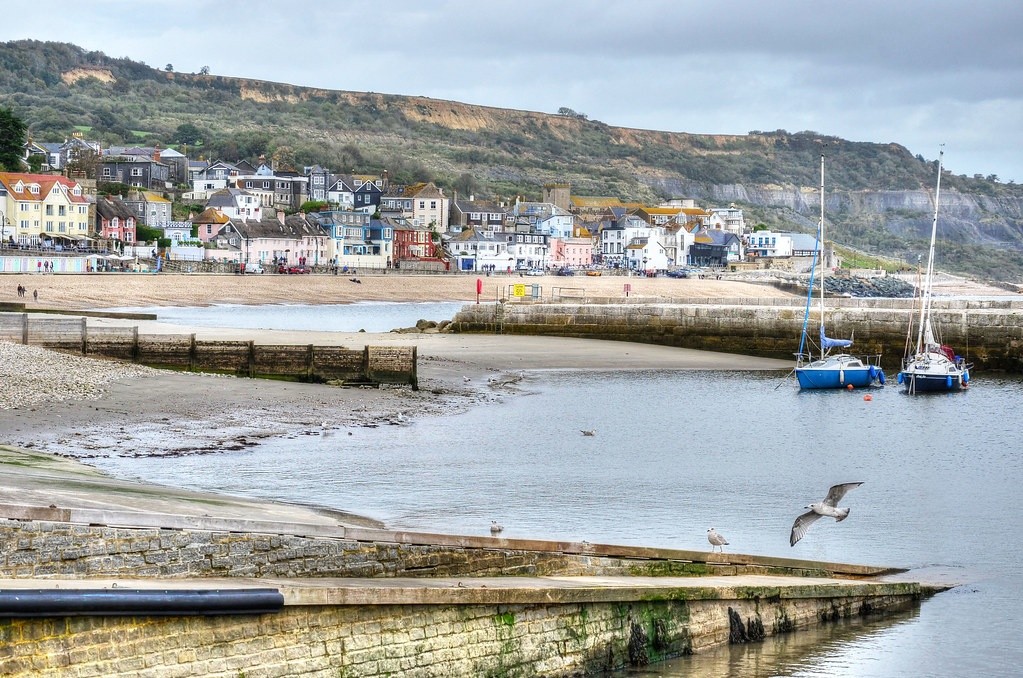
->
[87,254,135,266]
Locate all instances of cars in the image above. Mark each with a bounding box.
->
[558,268,574,277]
[587,269,602,277]
[529,269,546,276]
[245,263,265,273]
[279,264,310,275]
[643,265,726,281]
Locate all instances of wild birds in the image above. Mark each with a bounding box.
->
[789,482,865,547]
[580,428,597,437]
[462,375,471,386]
[490,520,504,534]
[321,420,331,435]
[707,527,730,554]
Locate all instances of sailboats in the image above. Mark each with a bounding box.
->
[900,142,976,395]
[776,153,883,391]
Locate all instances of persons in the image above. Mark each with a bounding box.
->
[630,266,722,280]
[9,238,88,254]
[531,263,590,270]
[18,284,27,297]
[272,256,349,275]
[385,259,400,268]
[34,289,37,301]
[479,262,496,276]
[97,260,141,271]
[38,260,54,272]
[234,264,245,274]
